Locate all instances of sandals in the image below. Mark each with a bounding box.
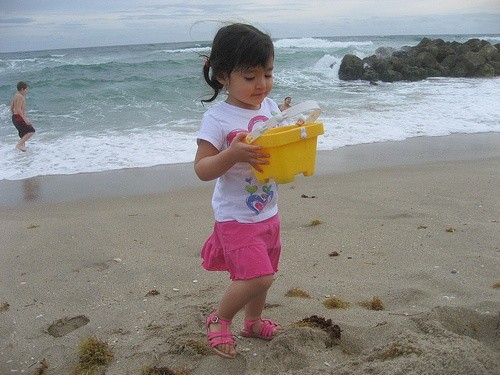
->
[239,320,284,340]
[206,311,236,358]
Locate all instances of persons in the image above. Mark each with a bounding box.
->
[189,20,308,361]
[9,81,36,152]
[275,97,294,113]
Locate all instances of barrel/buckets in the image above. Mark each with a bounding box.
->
[243,122,324,184]
